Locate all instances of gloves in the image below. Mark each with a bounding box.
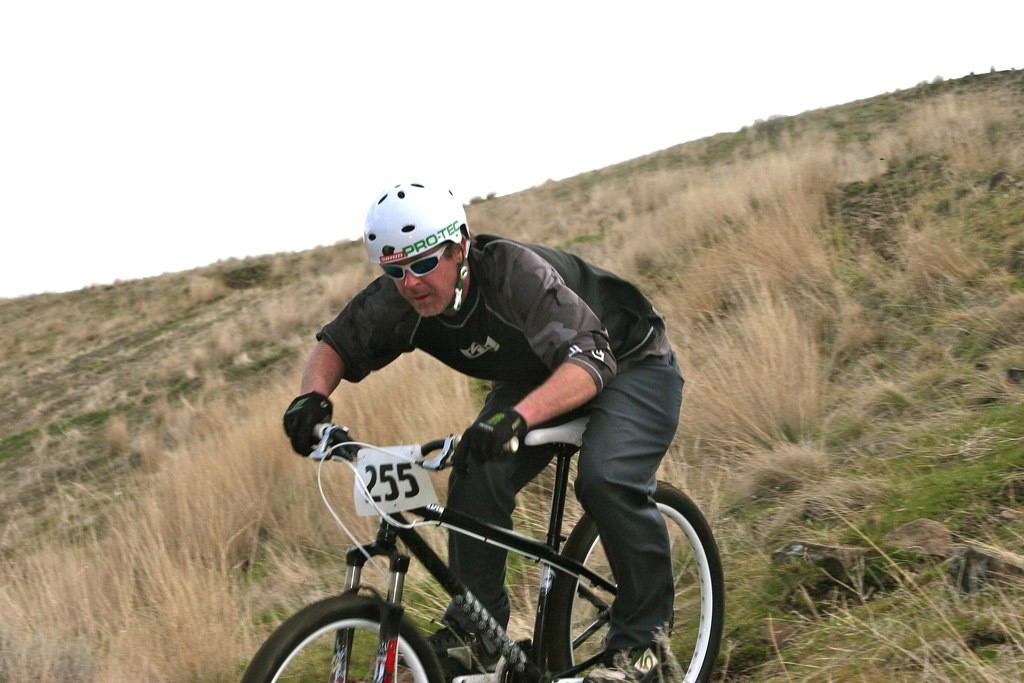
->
[450,408,528,481]
[283,391,333,458]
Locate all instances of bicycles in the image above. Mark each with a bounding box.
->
[227,414,724,683]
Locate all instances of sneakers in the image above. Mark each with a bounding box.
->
[397,627,504,677]
[584,644,673,683]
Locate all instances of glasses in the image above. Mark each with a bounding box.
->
[379,243,453,281]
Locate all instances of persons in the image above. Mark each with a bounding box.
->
[284,174,687,675]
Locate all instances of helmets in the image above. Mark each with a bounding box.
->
[361,181,471,264]
